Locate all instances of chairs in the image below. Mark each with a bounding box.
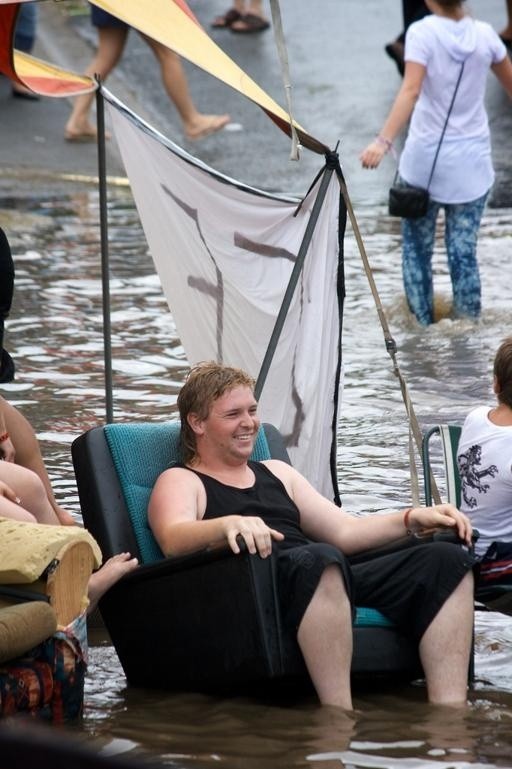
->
[0,494,102,726]
[71,423,479,709]
[422,427,512,612]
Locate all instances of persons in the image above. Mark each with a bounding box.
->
[1,460,140,618]
[148,364,481,713]
[2,1,49,104]
[210,1,273,39]
[382,0,444,79]
[355,0,510,330]
[0,225,77,532]
[447,335,511,616]
[58,1,235,153]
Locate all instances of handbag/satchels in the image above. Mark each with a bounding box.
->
[386,185,429,218]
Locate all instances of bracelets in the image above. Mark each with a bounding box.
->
[1,432,11,443]
[371,132,395,157]
[403,508,416,532]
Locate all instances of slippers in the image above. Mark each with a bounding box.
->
[188,113,229,141]
[64,123,109,141]
[212,9,268,33]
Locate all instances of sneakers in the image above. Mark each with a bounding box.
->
[12,83,39,100]
[386,41,405,75]
